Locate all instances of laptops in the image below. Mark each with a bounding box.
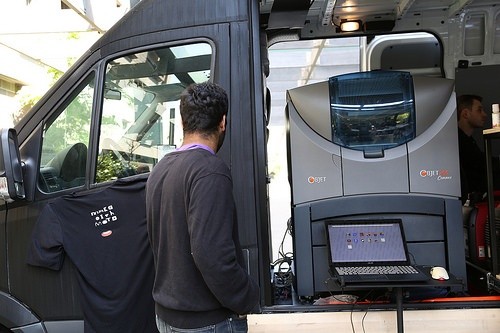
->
[325,219,427,283]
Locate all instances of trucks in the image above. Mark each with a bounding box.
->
[1,0,500,331]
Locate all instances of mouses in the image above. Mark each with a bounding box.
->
[430,266,449,281]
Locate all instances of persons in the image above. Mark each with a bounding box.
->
[144,80,259,333]
[457,94,488,291]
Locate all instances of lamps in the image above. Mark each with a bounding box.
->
[338,18,363,34]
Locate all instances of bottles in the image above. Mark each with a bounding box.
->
[492,104,500,129]
